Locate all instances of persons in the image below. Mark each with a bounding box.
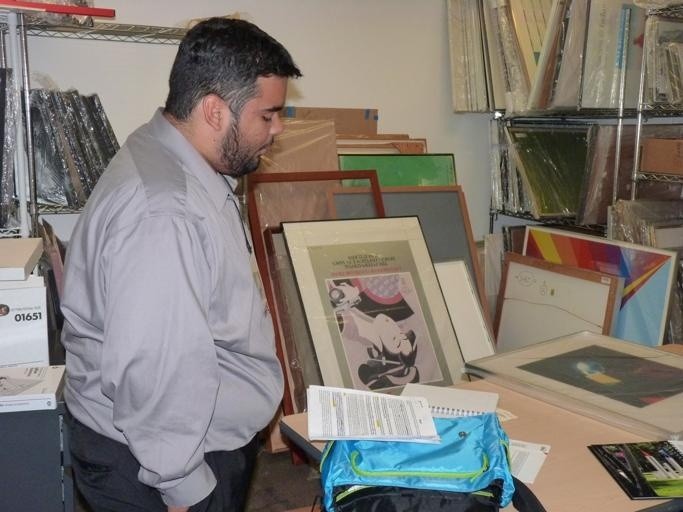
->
[326,273,418,389]
[56,19,303,507]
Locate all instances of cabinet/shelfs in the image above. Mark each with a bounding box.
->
[481,0,682,250]
[5,10,252,232]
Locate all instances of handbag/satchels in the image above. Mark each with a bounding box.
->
[310,413,544,512]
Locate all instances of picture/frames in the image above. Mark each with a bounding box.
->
[281,214,472,395]
[522,224,678,349]
[247,104,459,190]
[487,255,624,350]
[465,329,683,439]
[327,185,499,357]
[245,168,385,467]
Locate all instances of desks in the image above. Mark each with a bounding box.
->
[279,341,683,512]
